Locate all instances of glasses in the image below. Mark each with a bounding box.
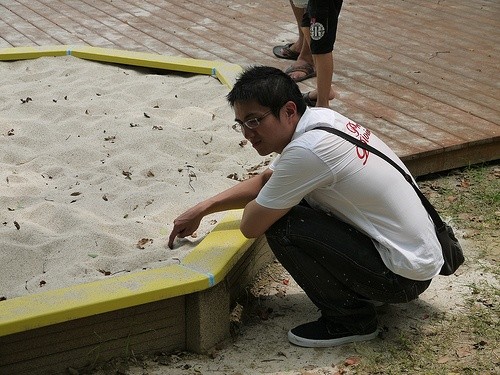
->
[232,101,288,133]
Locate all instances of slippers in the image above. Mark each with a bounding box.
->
[301,92,316,106]
[285,62,317,82]
[273,43,299,59]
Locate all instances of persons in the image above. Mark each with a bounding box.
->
[281,0,345,108]
[168,65,446,347]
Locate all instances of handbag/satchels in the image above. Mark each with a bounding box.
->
[434,223,465,276]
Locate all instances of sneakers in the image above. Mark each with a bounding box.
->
[288,310,380,347]
